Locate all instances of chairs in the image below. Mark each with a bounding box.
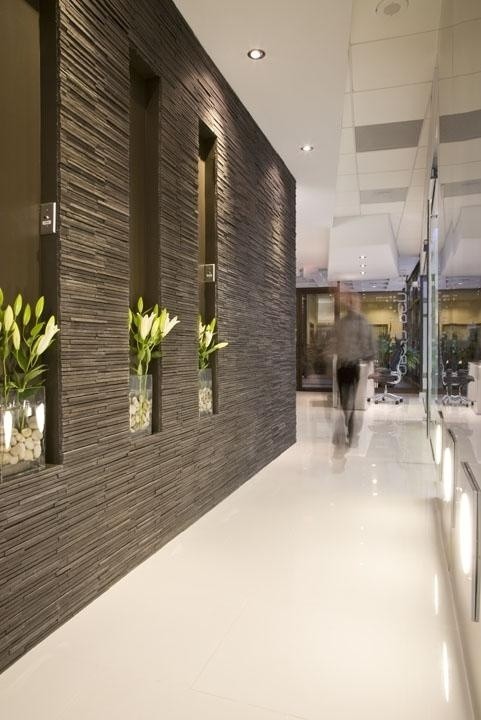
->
[440,348,474,407]
[368,348,405,406]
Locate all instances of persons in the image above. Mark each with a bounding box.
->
[319,293,376,449]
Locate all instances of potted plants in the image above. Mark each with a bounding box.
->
[0,288,61,482]
[127,295,182,439]
[198,314,229,417]
[310,329,330,375]
